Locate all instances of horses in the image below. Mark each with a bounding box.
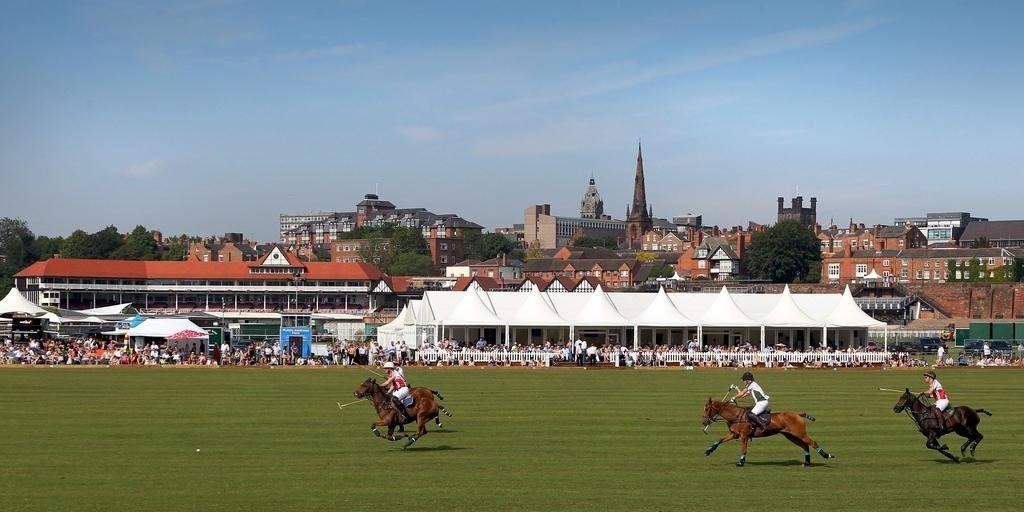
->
[353,377,454,450]
[697,397,835,468]
[893,387,992,462]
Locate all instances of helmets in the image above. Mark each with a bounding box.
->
[382,362,395,369]
[740,372,754,380]
[923,370,936,379]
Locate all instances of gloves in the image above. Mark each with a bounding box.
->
[730,384,738,390]
[730,397,736,403]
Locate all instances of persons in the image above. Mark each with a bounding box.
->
[376,361,410,423]
[415,337,487,366]
[307,338,407,366]
[936,344,946,366]
[575,337,669,367]
[391,359,404,378]
[488,337,572,366]
[827,344,928,368]
[917,370,950,436]
[210,341,304,366]
[766,340,823,368]
[958,341,1022,367]
[729,372,770,434]
[945,355,954,366]
[2,336,207,366]
[670,338,761,367]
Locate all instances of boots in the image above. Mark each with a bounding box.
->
[748,413,768,434]
[934,408,946,432]
[391,396,410,423]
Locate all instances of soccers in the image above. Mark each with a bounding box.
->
[196,449,201,453]
